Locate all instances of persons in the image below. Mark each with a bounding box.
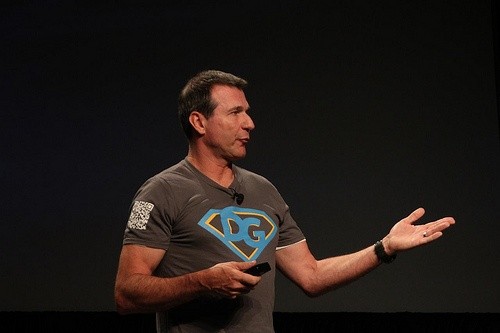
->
[113,71,457,333]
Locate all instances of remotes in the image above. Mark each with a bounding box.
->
[241,262,271,277]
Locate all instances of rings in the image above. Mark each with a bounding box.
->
[423,232,427,238]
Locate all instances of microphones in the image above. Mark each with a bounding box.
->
[230,188,244,204]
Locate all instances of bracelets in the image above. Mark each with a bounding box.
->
[374,240,398,265]
